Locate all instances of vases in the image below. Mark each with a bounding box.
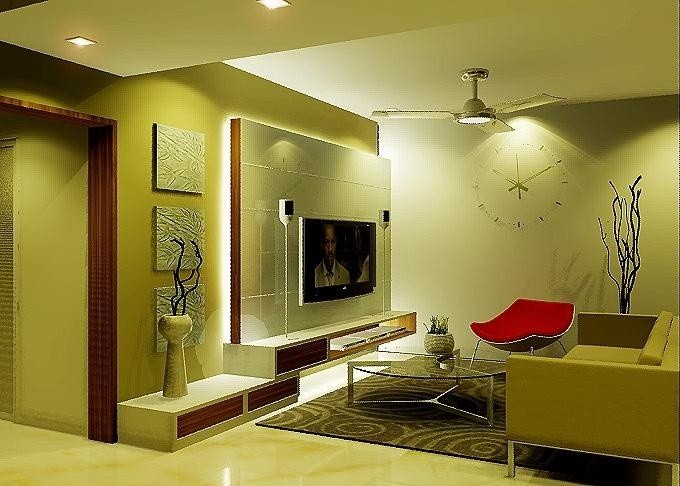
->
[157,316,192,402]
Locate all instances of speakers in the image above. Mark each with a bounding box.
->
[278,198,294,227]
[379,209,390,230]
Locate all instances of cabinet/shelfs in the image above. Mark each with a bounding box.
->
[116,311,418,453]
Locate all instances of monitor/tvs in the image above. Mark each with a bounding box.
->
[298,216,376,307]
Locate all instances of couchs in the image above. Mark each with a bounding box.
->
[503,312,680,486]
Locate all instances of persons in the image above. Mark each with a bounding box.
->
[315,222,350,288]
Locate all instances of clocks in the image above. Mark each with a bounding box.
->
[471,141,569,228]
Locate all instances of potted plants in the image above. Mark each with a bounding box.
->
[422,314,454,354]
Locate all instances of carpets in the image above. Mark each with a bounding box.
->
[255,356,554,473]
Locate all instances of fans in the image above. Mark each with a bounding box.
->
[371,68,568,136]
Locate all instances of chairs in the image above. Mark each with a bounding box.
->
[468,299,576,368]
